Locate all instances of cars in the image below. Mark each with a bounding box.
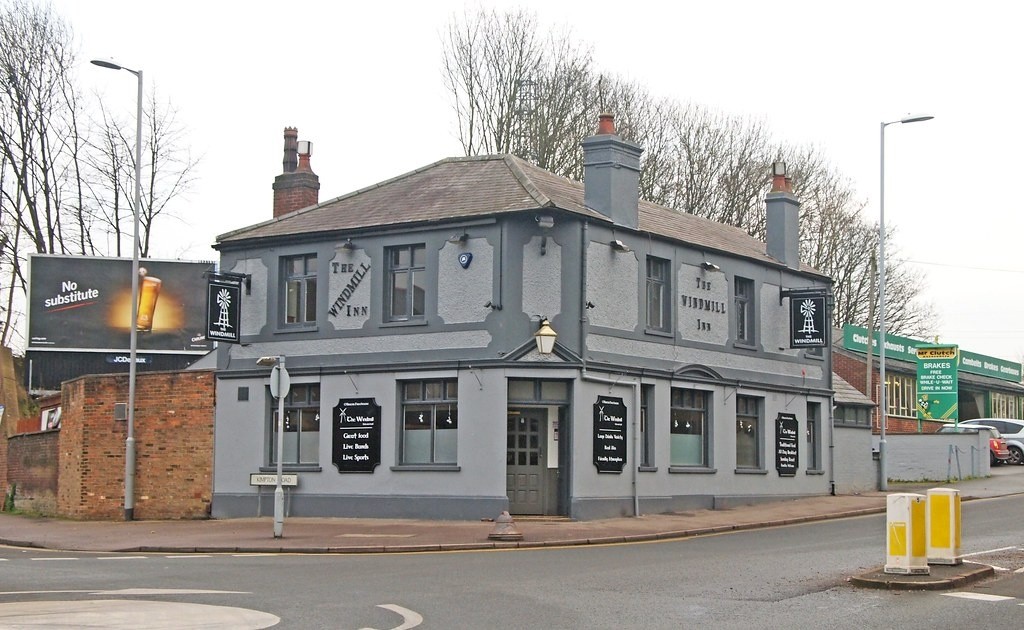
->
[961,418,1024,465]
[935,424,1011,467]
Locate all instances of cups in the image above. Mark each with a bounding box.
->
[137,277,162,334]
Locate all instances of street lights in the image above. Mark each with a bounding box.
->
[878,115,935,491]
[90,59,143,522]
